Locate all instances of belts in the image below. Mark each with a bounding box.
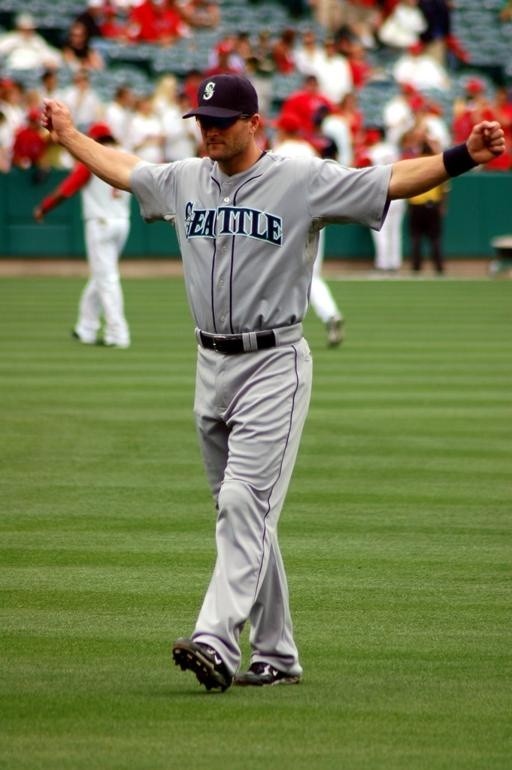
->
[201,331,276,354]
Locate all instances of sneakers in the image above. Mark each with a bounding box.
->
[173,638,231,691]
[236,662,299,686]
[326,315,342,348]
[73,329,128,348]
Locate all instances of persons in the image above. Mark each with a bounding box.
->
[0,0,512,179]
[410,140,449,271]
[42,74,506,697]
[33,122,130,348]
[271,123,345,345]
[355,125,411,269]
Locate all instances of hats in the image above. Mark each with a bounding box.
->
[183,73,258,118]
